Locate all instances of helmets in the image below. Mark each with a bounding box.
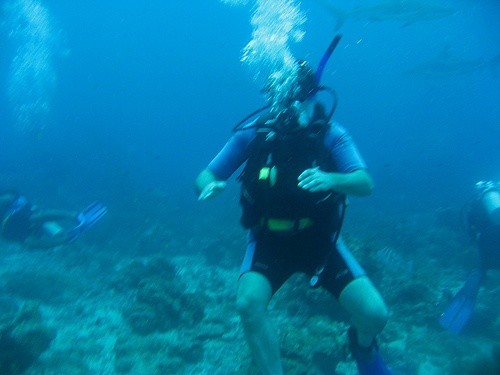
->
[471,181,499,204]
[1,193,37,241]
[267,59,317,104]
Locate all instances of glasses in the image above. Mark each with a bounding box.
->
[294,89,316,103]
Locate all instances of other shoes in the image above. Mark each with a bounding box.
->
[346,327,388,375]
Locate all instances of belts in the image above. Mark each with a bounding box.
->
[261,216,312,232]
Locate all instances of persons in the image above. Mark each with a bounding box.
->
[1,193,85,250]
[467,178,500,269]
[192,62,391,375]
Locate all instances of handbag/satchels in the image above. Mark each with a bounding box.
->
[259,163,341,206]
